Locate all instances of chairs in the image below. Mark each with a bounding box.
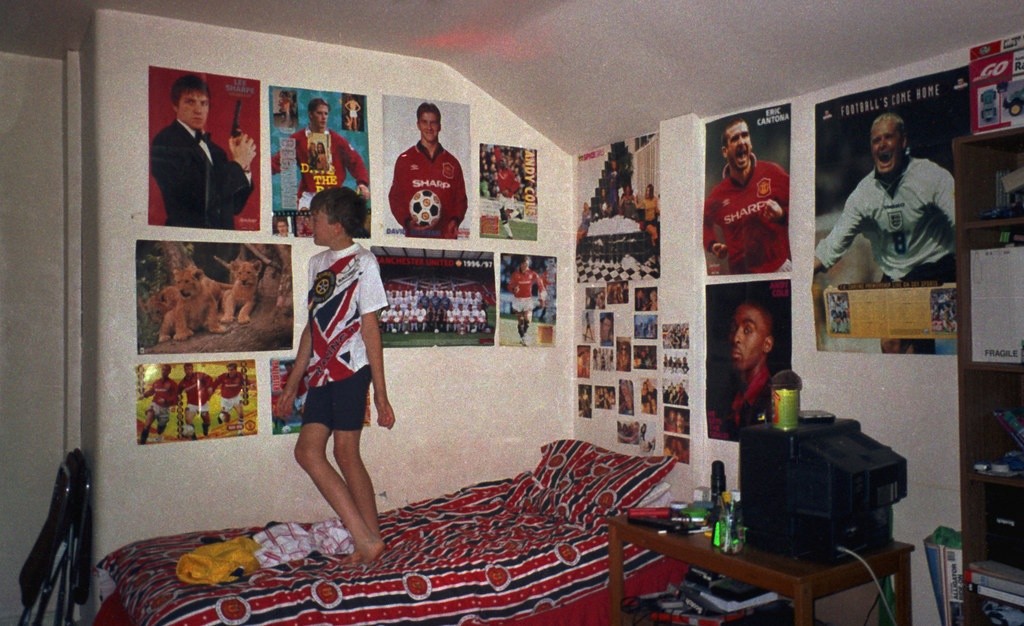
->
[17,446,95,626]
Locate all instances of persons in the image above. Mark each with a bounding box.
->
[150,74,258,230]
[381,282,487,331]
[929,291,954,328]
[507,258,544,346]
[532,270,556,322]
[814,111,955,354]
[705,301,774,441]
[276,186,395,565]
[275,217,288,238]
[493,145,522,239]
[579,283,690,463]
[278,91,291,116]
[279,362,308,414]
[576,160,660,258]
[702,118,789,275]
[389,103,469,240]
[345,97,361,130]
[271,98,369,210]
[136,363,250,445]
[830,295,849,331]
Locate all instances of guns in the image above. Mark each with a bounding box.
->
[230,100,242,137]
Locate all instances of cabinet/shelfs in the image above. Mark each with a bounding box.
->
[951,127,1023,626]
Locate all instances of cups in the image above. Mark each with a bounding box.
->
[711,461,727,500]
[719,510,737,554]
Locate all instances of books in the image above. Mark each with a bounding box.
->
[963,559,1024,608]
[699,581,777,613]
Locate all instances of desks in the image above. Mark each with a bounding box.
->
[606,516,915,625]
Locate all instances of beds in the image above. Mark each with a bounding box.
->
[97,481,686,626]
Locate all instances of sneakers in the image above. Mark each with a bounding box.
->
[519,336,528,346]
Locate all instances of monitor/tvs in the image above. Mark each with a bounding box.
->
[740,418,908,563]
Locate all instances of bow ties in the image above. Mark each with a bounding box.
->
[195,128,212,144]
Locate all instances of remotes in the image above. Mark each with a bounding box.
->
[628,516,687,532]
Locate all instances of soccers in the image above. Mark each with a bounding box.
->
[434,329,439,334]
[408,189,442,227]
[391,328,397,333]
[181,424,195,437]
[404,331,409,335]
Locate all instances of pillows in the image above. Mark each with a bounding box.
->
[511,439,679,515]
